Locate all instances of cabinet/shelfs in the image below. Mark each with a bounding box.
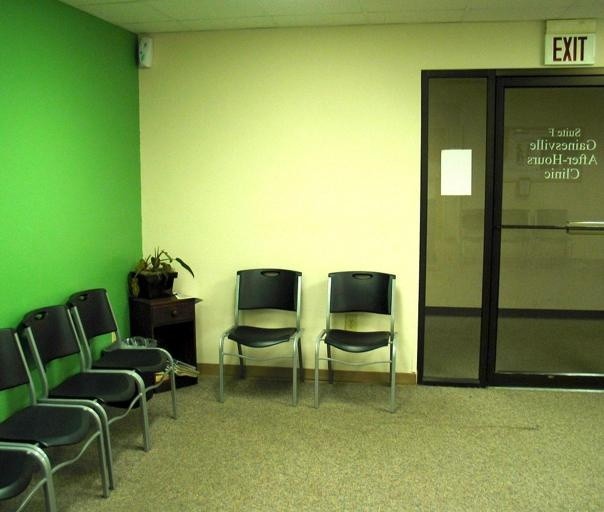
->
[128,297,199,394]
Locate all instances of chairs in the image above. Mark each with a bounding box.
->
[19,304,150,490]
[67,287,176,451]
[219,268,398,413]
[3,328,109,510]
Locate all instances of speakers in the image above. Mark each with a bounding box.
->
[139,37,153,67]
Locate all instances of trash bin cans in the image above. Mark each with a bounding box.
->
[101,336,157,409]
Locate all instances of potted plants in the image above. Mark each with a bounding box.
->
[126,246,196,299]
